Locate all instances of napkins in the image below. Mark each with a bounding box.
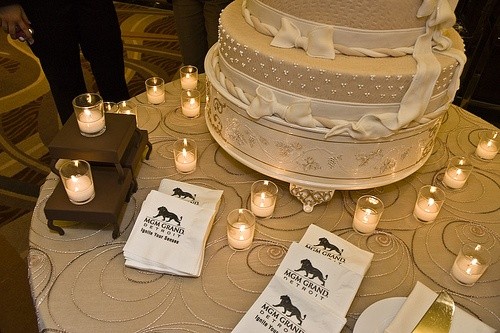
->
[123,179,224,277]
[383,281,496,333]
[231,224,374,333]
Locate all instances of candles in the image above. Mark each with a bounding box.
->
[354,208,379,233]
[176,148,196,173]
[183,99,200,117]
[66,174,93,202]
[414,198,438,221]
[443,168,466,189]
[474,132,498,161]
[230,224,252,249]
[252,192,275,218]
[450,255,482,284]
[78,107,105,135]
[182,73,198,89]
[148,85,165,105]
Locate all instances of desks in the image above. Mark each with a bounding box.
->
[28,73,500,333]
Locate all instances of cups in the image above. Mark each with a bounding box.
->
[181,89,200,119]
[226,208,256,251]
[475,130,500,161]
[173,139,197,174]
[180,66,198,90]
[118,101,138,127]
[412,185,445,224]
[145,77,165,105]
[443,156,473,189]
[250,180,278,219]
[352,195,384,235]
[72,93,106,137]
[450,243,491,287]
[104,102,119,114]
[59,160,96,205]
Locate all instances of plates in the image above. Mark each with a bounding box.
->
[352,297,407,333]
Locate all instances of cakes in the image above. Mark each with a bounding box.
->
[214,0,466,121]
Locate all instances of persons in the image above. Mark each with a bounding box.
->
[0,0,130,125]
[174,0,232,74]
